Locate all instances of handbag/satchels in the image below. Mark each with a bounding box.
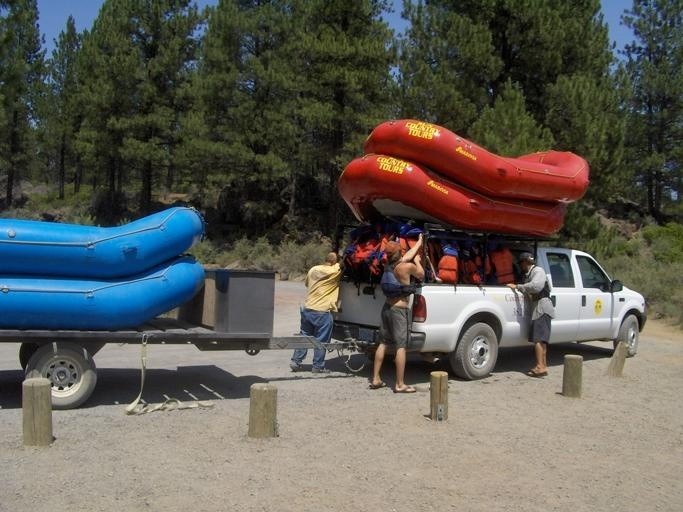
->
[380,270,403,298]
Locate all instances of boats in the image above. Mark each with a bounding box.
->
[0,255,205,330]
[0,205,205,278]
[338,152,572,239]
[363,119,590,204]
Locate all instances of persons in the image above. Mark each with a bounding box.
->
[369,230,427,393]
[504,251,556,377]
[288,252,347,373]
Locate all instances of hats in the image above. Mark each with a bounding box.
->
[517,252,536,266]
[384,241,401,258]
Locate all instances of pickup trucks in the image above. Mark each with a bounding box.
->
[330,245,648,383]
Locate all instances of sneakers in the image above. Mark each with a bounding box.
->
[290,359,306,372]
[312,366,330,374]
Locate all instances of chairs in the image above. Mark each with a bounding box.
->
[550,264,584,287]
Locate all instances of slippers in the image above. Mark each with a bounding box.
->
[528,369,547,377]
[393,386,416,394]
[370,380,387,389]
